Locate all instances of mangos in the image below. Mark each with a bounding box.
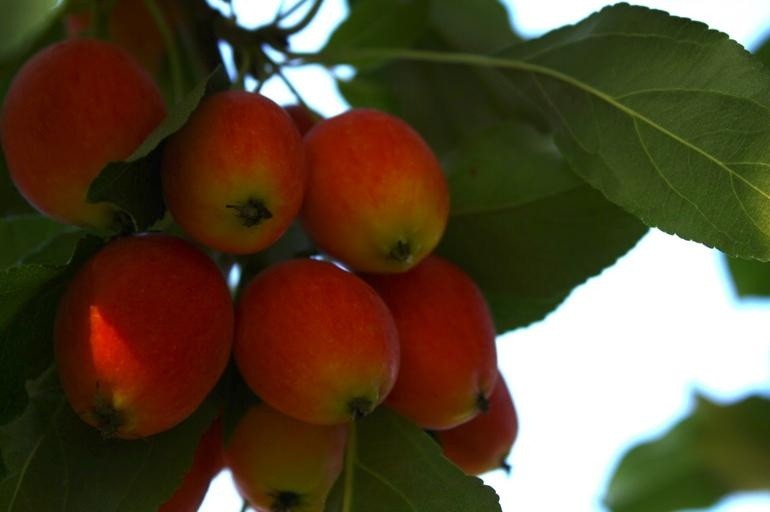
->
[2,0,516,512]
